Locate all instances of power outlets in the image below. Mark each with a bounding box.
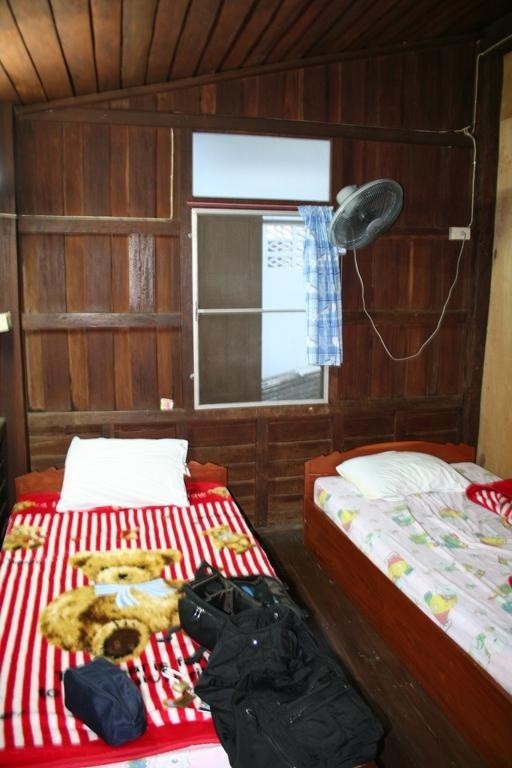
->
[449,227,471,241]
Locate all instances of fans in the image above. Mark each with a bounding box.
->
[329,180,403,251]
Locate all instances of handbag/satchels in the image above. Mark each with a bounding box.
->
[63,659,149,747]
[176,560,305,652]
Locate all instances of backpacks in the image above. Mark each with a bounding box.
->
[194,607,385,768]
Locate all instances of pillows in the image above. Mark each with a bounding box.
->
[55,441,193,515]
[334,448,473,499]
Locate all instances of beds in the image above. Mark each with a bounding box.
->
[298,440,512,766]
[8,455,296,767]
